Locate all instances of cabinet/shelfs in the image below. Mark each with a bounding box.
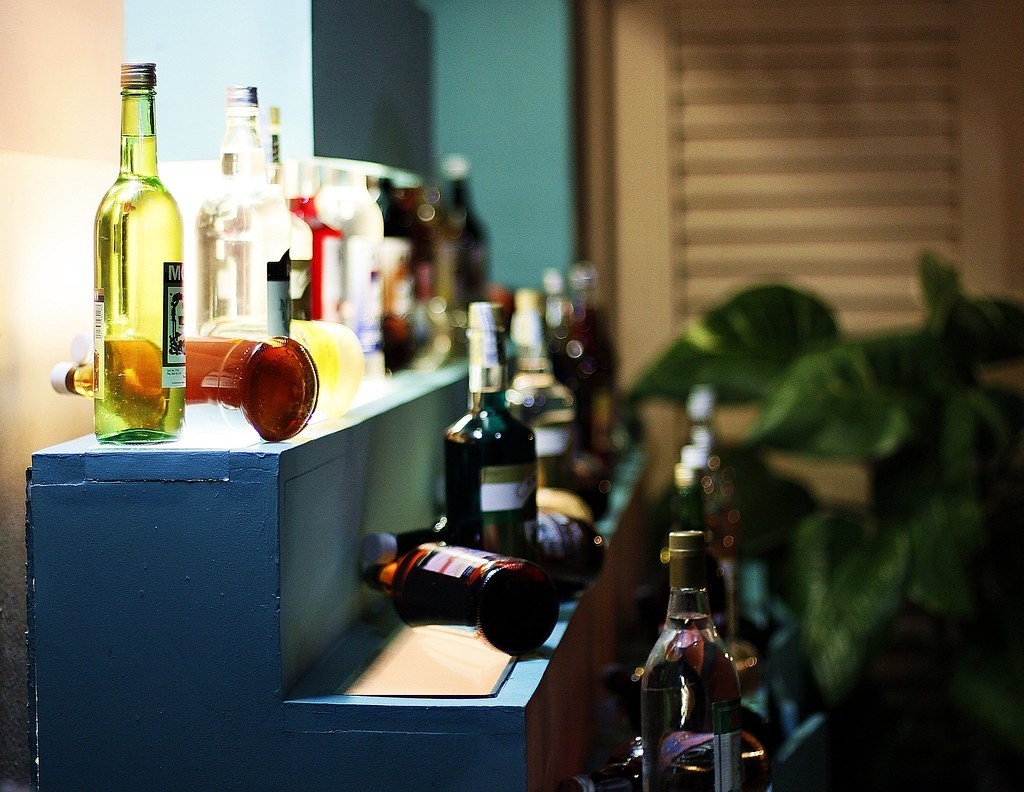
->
[26,355,648,792]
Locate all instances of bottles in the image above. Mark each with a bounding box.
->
[313,167,386,400]
[201,318,364,417]
[564,730,770,792]
[401,199,431,352]
[444,302,537,562]
[656,464,726,642]
[506,288,576,486]
[558,264,616,467]
[195,85,291,340]
[443,157,488,357]
[51,337,319,443]
[370,177,415,370]
[93,63,185,445]
[368,542,561,656]
[365,514,605,589]
[642,532,743,792]
[283,160,347,323]
[260,102,311,319]
